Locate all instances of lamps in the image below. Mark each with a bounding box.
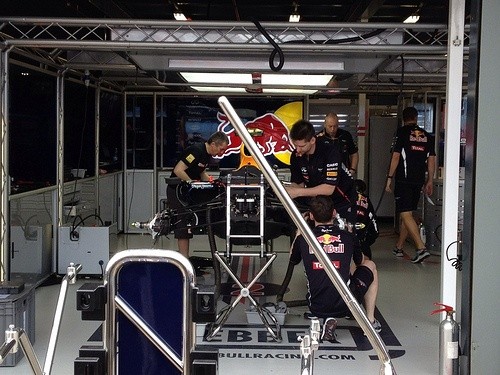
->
[173,2,188,21]
[403,2,423,24]
[289,2,300,23]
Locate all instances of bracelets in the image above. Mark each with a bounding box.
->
[388,176,393,178]
[350,168,356,171]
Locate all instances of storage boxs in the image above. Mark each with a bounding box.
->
[0,288,35,367]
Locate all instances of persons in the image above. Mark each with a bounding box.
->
[289,195,381,341]
[276,112,379,301]
[385,107,435,263]
[166,132,229,269]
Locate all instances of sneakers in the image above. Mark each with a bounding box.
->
[410,248,430,263]
[321,318,338,341]
[364,318,381,337]
[392,246,404,257]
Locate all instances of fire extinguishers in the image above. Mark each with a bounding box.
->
[431,304,460,375]
